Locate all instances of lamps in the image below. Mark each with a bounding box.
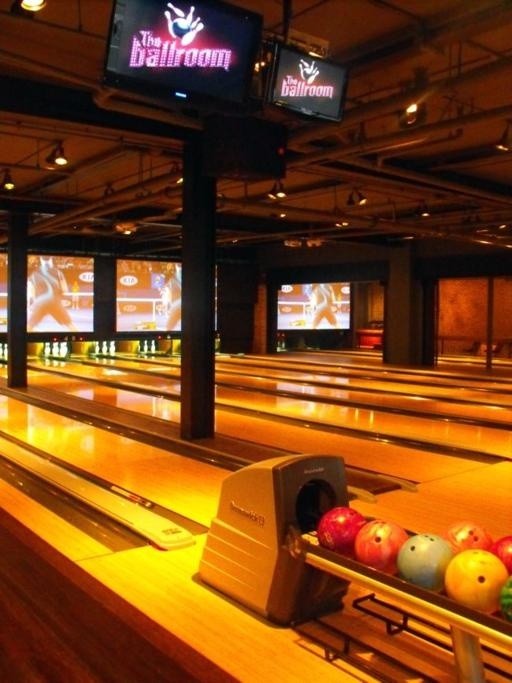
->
[335,217,350,228]
[495,123,511,152]
[3,169,16,191]
[355,190,368,207]
[267,186,277,200]
[276,182,288,199]
[53,141,68,166]
[20,0,47,13]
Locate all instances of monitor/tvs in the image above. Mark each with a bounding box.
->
[104,0,265,114]
[268,36,348,124]
